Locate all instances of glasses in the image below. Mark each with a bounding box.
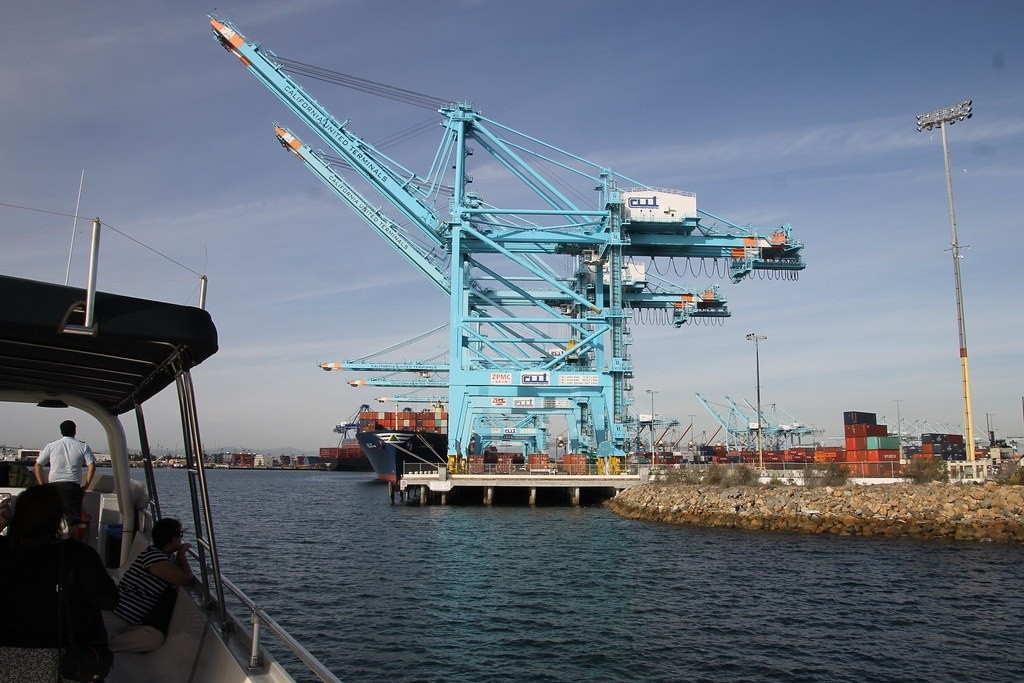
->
[172,531,183,537]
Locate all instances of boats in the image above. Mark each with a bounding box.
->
[332,404,449,488]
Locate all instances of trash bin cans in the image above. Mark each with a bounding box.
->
[104,523,123,570]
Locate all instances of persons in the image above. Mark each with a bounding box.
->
[34,420,97,541]
[0,485,122,683]
[101,518,193,651]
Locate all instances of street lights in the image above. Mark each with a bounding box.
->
[745,331,768,471]
[915,98,977,462]
[645,389,660,465]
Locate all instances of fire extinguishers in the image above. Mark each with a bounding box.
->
[78,512,91,545]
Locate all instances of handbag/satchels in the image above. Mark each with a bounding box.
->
[60,642,113,680]
